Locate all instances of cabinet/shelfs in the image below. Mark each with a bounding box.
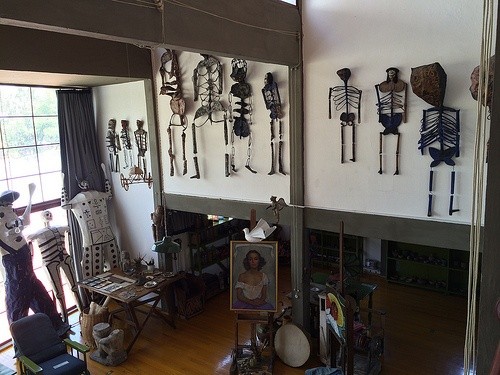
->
[232,312,275,375]
[310,229,363,268]
[187,217,246,301]
[386,241,482,297]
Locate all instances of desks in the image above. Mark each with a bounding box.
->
[76,263,184,354]
[287,280,377,325]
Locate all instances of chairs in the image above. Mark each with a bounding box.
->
[10,313,91,375]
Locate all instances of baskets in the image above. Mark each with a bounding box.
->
[82,306,109,346]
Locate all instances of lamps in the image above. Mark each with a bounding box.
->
[154,195,180,253]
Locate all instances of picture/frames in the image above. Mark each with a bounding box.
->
[230,240,278,312]
